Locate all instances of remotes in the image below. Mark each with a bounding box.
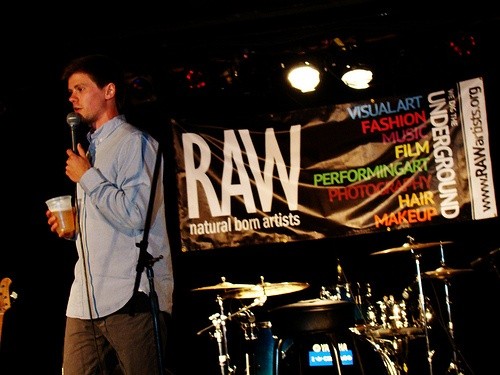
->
[336,264,345,288]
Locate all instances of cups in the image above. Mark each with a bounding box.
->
[45,195,74,234]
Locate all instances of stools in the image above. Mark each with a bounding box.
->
[269,298,369,375]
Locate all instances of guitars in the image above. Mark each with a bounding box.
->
[0,276,18,340]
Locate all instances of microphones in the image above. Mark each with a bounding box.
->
[67,112,81,154]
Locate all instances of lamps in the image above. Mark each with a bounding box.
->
[284,47,375,93]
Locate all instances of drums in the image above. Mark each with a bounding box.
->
[365,297,432,338]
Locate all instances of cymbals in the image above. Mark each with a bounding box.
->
[373,240,453,256]
[224,281,310,299]
[423,266,474,279]
[192,282,254,292]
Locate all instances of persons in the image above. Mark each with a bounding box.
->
[44,58,174,375]
